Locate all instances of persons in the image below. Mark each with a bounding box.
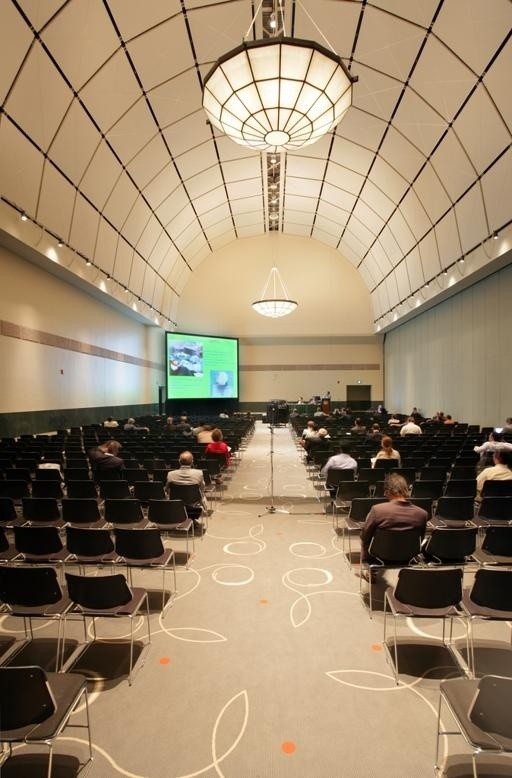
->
[104,416,119,427]
[368,422,383,438]
[123,418,136,433]
[331,408,351,420]
[323,391,331,402]
[90,439,123,479]
[177,416,190,432]
[206,429,232,467]
[351,418,367,434]
[163,417,175,430]
[301,420,315,435]
[476,444,511,498]
[166,451,205,506]
[314,407,329,418]
[298,428,330,461]
[473,432,511,453]
[322,440,358,487]
[410,407,422,418]
[359,474,428,571]
[371,436,401,470]
[288,408,301,422]
[400,417,423,438]
[499,417,511,436]
[430,411,454,429]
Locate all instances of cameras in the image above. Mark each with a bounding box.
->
[262,400,289,423]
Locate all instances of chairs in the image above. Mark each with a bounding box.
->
[63,468,90,481]
[152,468,175,482]
[448,570,511,675]
[372,480,385,496]
[482,480,510,496]
[423,526,481,567]
[148,499,197,570]
[35,468,62,480]
[59,496,100,526]
[1,480,29,498]
[383,566,471,687]
[103,498,147,529]
[64,525,124,576]
[122,468,149,482]
[31,480,64,498]
[0,497,15,526]
[472,527,512,566]
[5,467,32,480]
[169,483,209,536]
[0,523,9,558]
[0,560,73,671]
[408,497,433,531]
[66,480,97,499]
[333,481,369,535]
[319,467,356,515]
[343,496,389,569]
[20,496,60,524]
[410,480,443,498]
[290,410,494,481]
[197,459,223,498]
[428,496,475,529]
[113,526,177,619]
[13,523,65,567]
[445,478,477,498]
[0,665,92,778]
[99,479,131,499]
[202,469,217,510]
[62,571,152,686]
[356,523,424,618]
[477,496,512,526]
[0,412,254,467]
[435,676,511,778]
[133,481,166,501]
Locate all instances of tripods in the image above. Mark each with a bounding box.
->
[257,424,292,518]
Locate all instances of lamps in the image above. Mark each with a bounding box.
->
[253,264,297,317]
[193,1,359,164]
